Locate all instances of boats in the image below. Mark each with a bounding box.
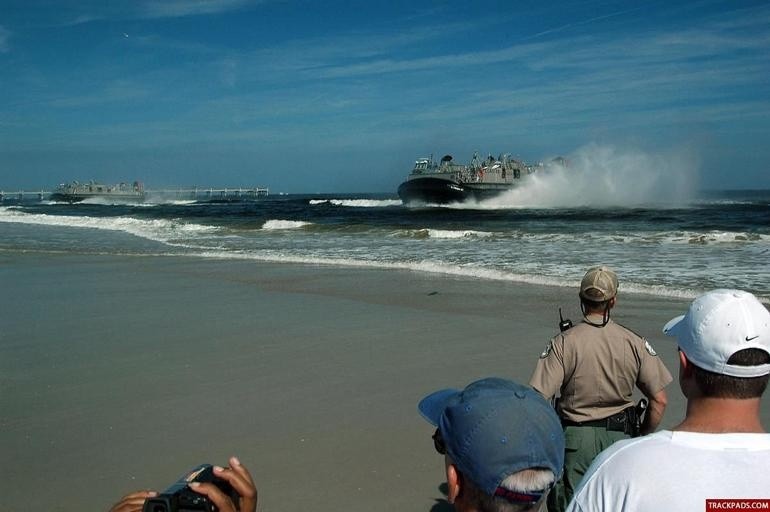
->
[398,151,568,206]
[53,179,146,206]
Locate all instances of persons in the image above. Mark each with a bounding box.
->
[110,457,258,512]
[564,287,769,512]
[529,267,675,512]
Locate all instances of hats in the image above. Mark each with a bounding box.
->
[662,288,770,376]
[580,265,620,303]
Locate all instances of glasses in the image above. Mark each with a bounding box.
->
[432,427,446,455]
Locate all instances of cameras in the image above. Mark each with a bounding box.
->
[143,463,240,512]
